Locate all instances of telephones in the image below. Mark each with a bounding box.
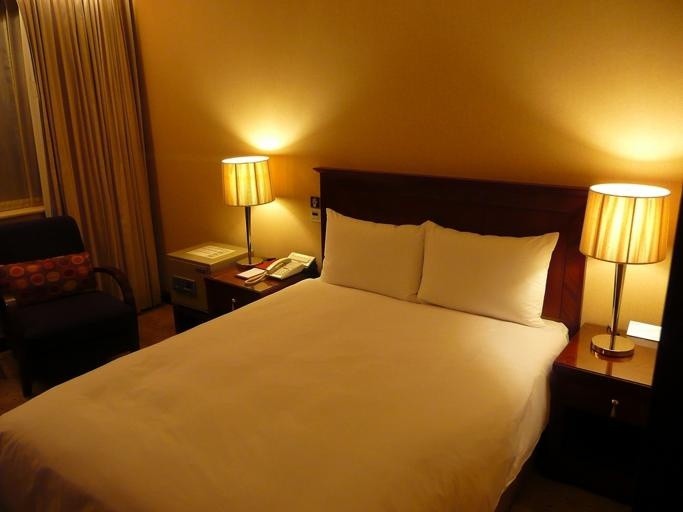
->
[264,256,307,280]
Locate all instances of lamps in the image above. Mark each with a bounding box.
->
[578,182,672,360]
[220,155,276,267]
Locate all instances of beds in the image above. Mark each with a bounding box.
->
[0,166,588,512]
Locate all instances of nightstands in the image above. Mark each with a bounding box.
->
[543,322,658,508]
[204,256,316,320]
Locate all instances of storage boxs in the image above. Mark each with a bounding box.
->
[166,241,255,317]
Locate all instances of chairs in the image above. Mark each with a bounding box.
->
[0,214,138,398]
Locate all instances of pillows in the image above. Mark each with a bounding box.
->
[321,207,559,332]
[0,251,97,303]
